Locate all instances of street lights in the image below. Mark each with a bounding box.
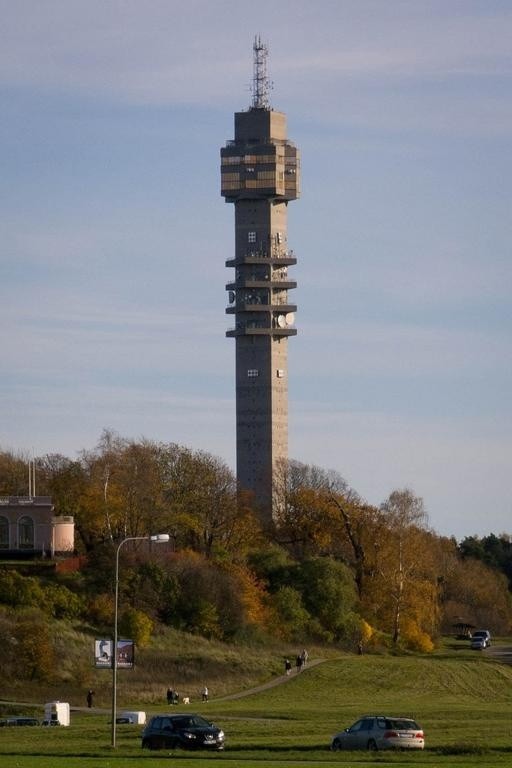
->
[111,532,170,750]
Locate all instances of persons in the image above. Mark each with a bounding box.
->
[284,658,291,677]
[295,654,302,674]
[167,686,174,706]
[99,640,110,662]
[301,647,308,667]
[87,688,96,708]
[357,640,363,655]
[201,685,209,703]
[173,687,180,705]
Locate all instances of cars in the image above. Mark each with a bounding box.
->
[141,713,224,751]
[470,629,491,649]
[332,715,425,751]
